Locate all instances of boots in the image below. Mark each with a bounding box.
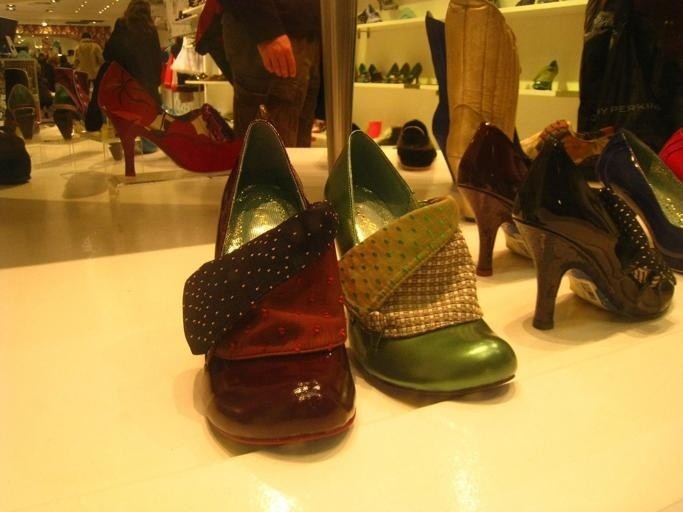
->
[425,0,521,222]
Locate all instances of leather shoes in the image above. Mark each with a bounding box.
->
[183,120,354,445]
[6,84,36,111]
[397,121,436,167]
[85,66,107,133]
[364,5,381,22]
[54,83,78,112]
[323,130,518,398]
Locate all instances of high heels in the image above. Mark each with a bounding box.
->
[99,62,239,176]
[357,63,421,84]
[533,60,558,91]
[378,0,398,10]
[108,135,156,160]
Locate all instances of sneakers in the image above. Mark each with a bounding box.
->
[0,126,31,184]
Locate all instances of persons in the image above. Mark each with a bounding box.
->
[101,0,163,107]
[76,33,105,95]
[192,2,323,146]
[38,52,56,91]
